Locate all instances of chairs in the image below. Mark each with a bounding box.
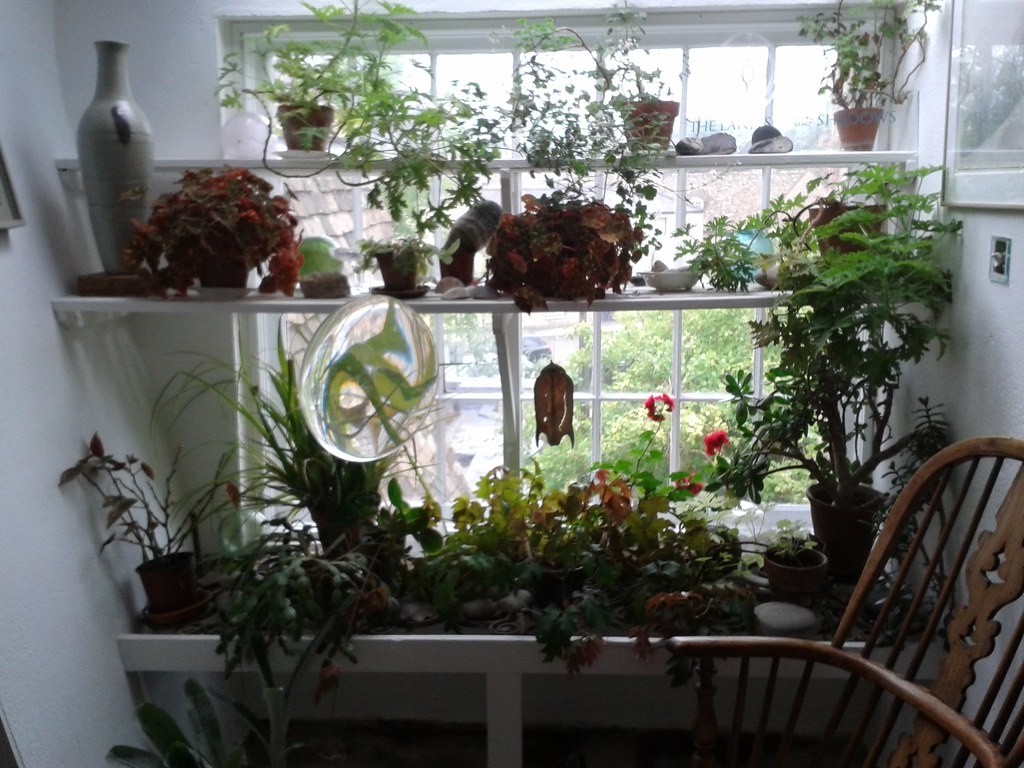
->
[665,436,1024,768]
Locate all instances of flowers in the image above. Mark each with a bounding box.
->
[128,163,303,299]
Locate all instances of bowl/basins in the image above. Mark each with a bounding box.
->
[642,269,699,292]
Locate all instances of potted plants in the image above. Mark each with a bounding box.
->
[208,0,504,235]
[706,250,959,581]
[511,470,631,604]
[508,0,679,167]
[56,429,238,630]
[139,319,410,558]
[759,518,825,603]
[799,0,943,153]
[355,236,461,298]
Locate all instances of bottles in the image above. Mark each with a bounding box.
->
[76,39,153,274]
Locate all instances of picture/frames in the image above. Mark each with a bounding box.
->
[938,0,1024,215]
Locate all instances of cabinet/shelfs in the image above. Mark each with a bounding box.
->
[47,150,947,669]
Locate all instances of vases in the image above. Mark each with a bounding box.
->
[182,229,251,290]
[76,40,154,276]
